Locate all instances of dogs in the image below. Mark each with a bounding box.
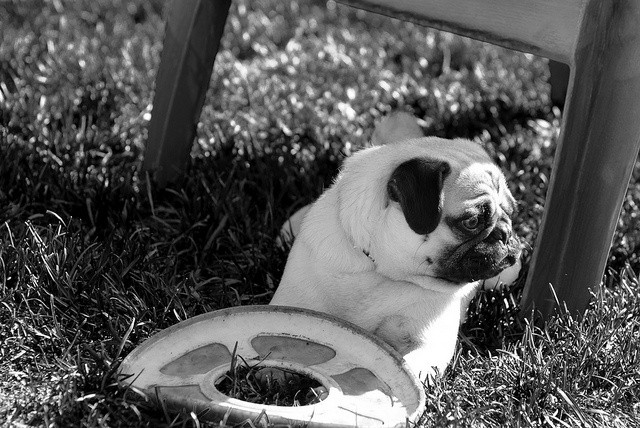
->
[267,110,522,389]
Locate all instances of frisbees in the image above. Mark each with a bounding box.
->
[112,303,426,425]
